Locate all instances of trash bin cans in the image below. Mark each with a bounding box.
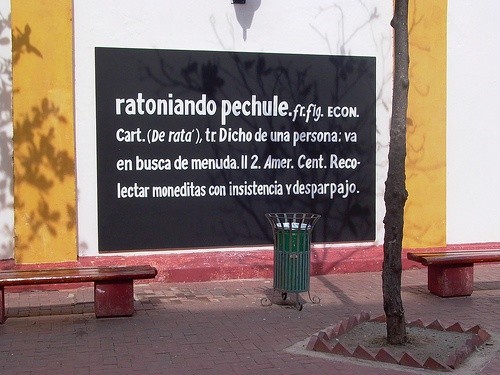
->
[260,211,321,311]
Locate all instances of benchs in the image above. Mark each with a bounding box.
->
[407,248,500,298]
[0,264,158,323]
